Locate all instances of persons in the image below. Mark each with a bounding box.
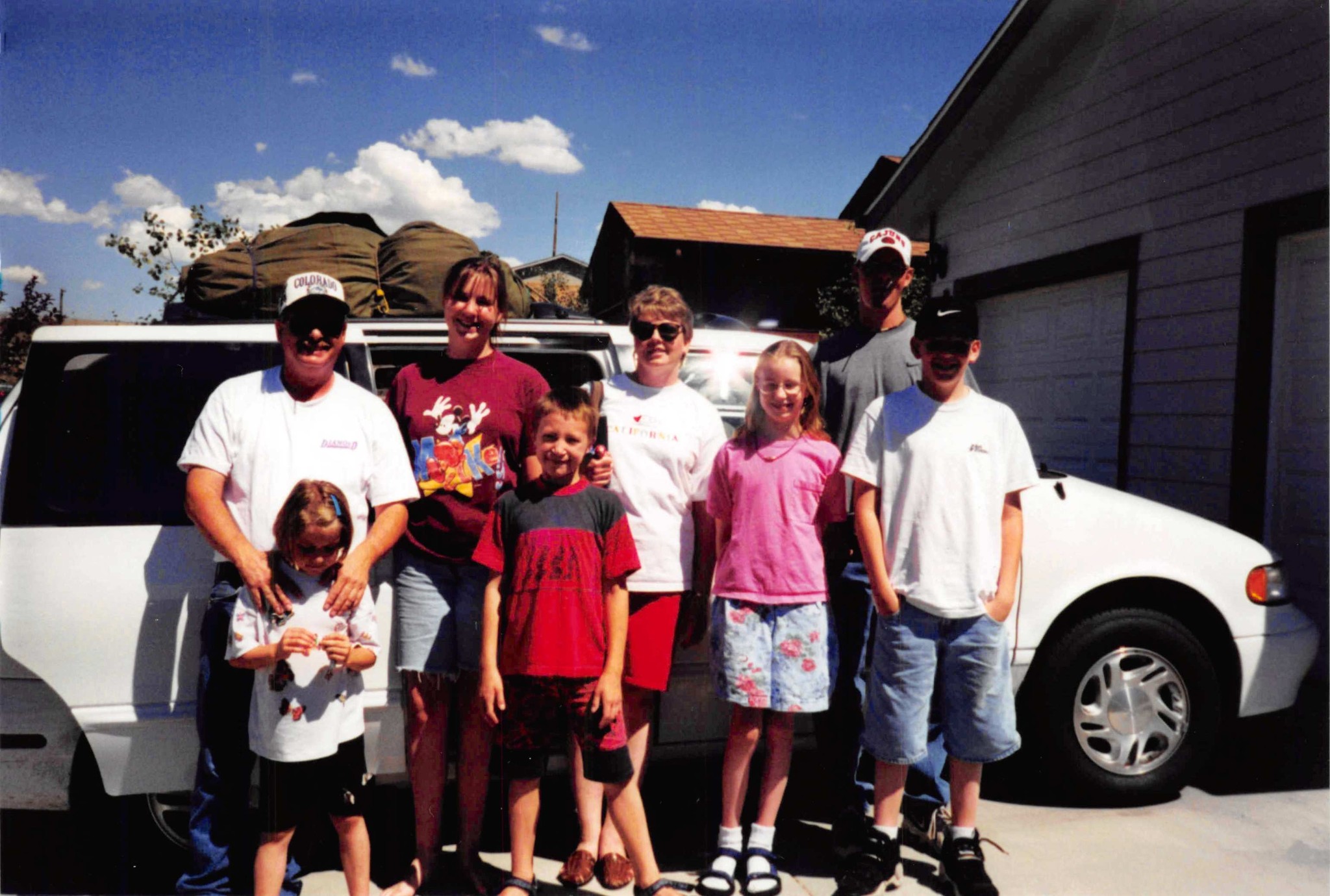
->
[807,226,982,877]
[554,285,729,891]
[470,385,688,896]
[224,479,379,896]
[830,295,1040,896]
[695,340,848,896]
[379,251,616,896]
[175,270,421,896]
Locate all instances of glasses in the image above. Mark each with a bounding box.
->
[285,312,343,337]
[861,262,905,278]
[635,321,683,342]
[925,340,968,355]
[758,381,807,396]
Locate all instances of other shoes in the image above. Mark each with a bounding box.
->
[597,853,634,888]
[559,850,596,885]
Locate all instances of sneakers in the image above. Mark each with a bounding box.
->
[941,828,999,896]
[838,828,903,896]
[901,806,952,862]
[831,816,874,866]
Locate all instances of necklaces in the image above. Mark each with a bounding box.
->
[858,312,908,332]
[751,426,805,462]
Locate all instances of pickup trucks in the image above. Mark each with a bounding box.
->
[0,315,1320,896]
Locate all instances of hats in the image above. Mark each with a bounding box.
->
[278,272,349,315]
[915,297,979,338]
[857,228,911,268]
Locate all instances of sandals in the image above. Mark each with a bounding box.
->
[743,848,782,896]
[634,878,672,896]
[504,875,538,896]
[696,847,741,896]
